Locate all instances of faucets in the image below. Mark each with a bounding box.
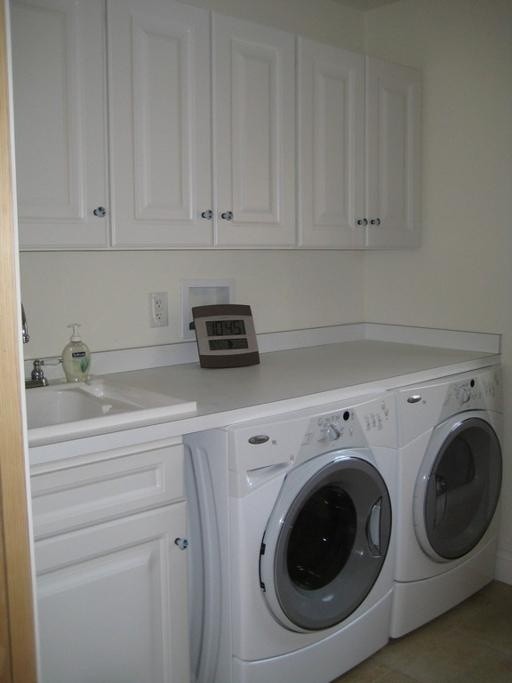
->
[30,358,51,385]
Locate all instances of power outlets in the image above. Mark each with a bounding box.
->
[151,291,169,326]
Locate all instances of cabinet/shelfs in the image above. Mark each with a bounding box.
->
[7,0,112,255]
[112,0,422,252]
[28,436,193,683]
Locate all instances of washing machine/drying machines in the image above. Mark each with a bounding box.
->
[314,319,506,641]
[96,346,392,683]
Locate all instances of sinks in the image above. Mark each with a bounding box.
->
[26,385,147,433]
